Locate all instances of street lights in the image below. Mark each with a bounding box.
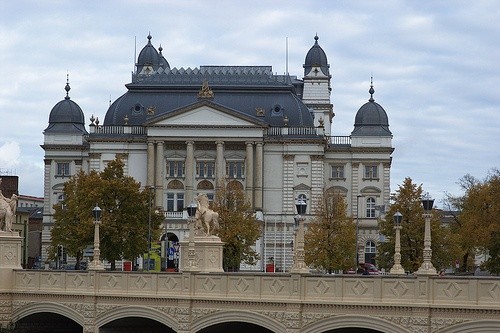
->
[87,203,105,271]
[181,201,201,273]
[416,191,436,275]
[147,186,156,272]
[386,208,406,274]
[291,195,308,270]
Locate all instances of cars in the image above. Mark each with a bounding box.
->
[358,262,381,276]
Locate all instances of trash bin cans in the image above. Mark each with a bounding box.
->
[44,262,49,270]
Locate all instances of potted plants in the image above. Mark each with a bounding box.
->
[121,250,133,271]
[268,257,275,272]
[167,261,176,272]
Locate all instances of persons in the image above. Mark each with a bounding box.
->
[358,264,370,275]
[439,271,445,279]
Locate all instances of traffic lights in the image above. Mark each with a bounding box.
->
[57,246,62,257]
